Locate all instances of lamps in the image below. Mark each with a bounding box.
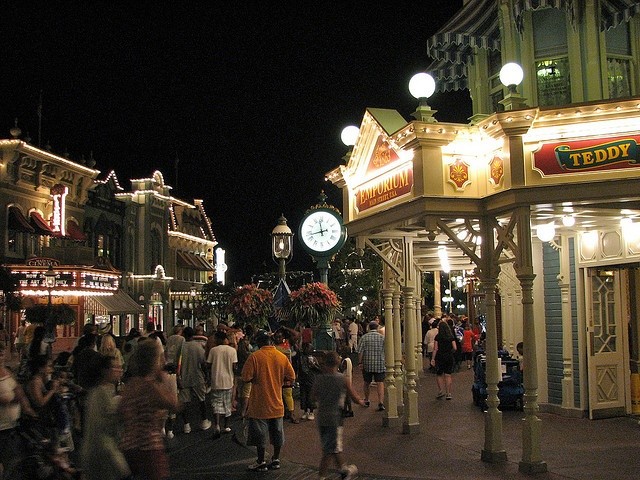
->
[498,61,524,101]
[407,73,437,113]
[340,125,361,156]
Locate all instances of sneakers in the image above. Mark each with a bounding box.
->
[183,422,192,434]
[247,460,268,472]
[225,427,232,432]
[445,393,453,399]
[363,399,370,408]
[378,402,386,411]
[202,418,212,430]
[342,463,359,478]
[301,411,309,419]
[215,425,220,436]
[308,412,315,420]
[435,389,446,398]
[268,456,280,470]
[167,430,175,439]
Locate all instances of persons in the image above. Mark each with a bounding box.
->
[441,312,456,326]
[516,342,524,372]
[356,322,386,411]
[430,321,458,400]
[454,314,485,371]
[308,353,370,480]
[300,318,365,354]
[0,324,178,480]
[173,323,241,435]
[273,325,299,424]
[296,343,321,419]
[241,329,296,471]
[422,314,440,358]
[366,316,382,332]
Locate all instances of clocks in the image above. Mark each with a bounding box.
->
[297,207,346,256]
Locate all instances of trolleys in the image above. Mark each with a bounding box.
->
[472,350,526,413]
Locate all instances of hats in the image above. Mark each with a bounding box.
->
[98,323,112,334]
[369,320,379,330]
